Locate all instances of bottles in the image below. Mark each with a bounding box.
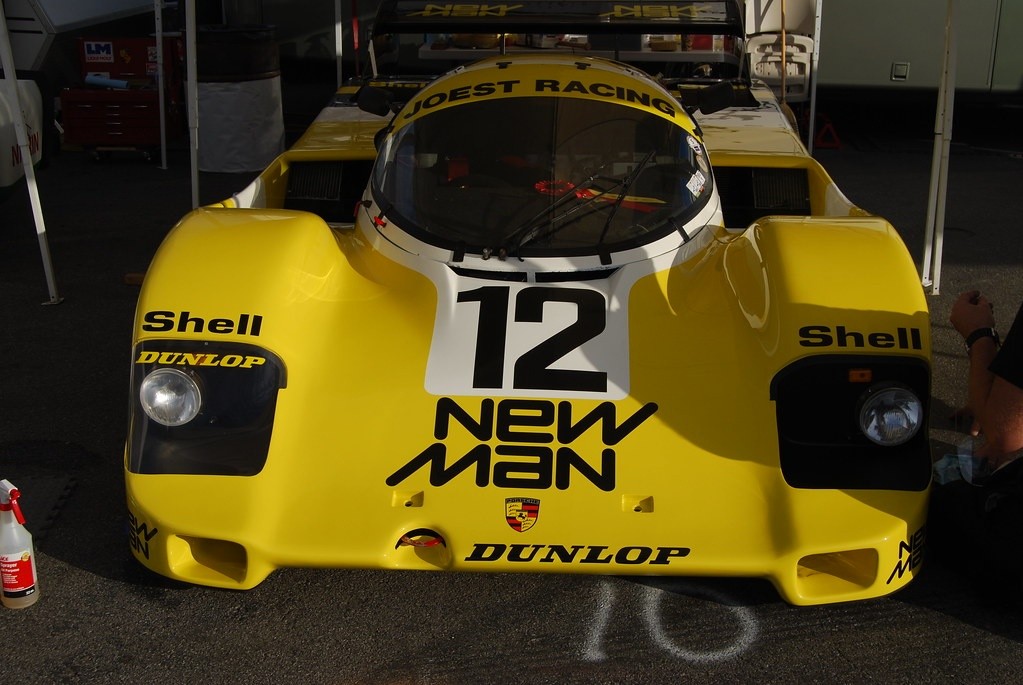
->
[0,479,40,610]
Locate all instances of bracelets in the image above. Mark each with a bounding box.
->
[964,328,1002,356]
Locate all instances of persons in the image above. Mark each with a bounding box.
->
[948,289,1022,454]
